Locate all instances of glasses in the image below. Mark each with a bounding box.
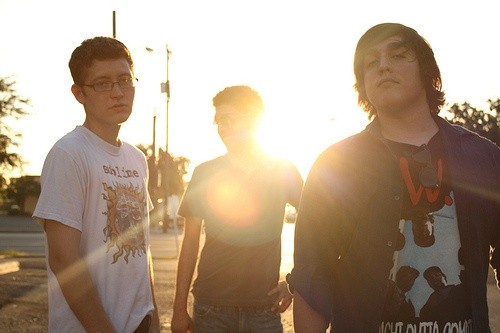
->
[81,76,139,93]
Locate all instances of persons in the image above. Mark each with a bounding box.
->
[170,85,304,333]
[35,37,161,333]
[293,22,500,333]
